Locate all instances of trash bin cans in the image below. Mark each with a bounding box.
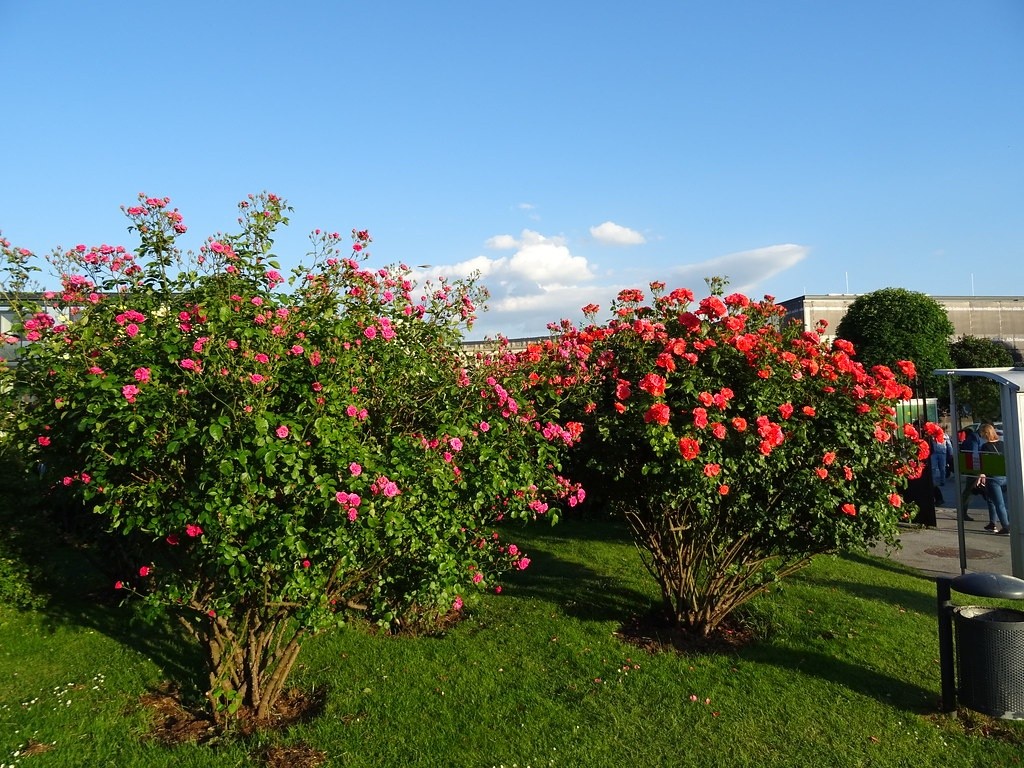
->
[935,571,1024,722]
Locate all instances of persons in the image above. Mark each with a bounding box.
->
[913,418,1010,536]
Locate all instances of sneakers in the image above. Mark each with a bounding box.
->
[984,523,998,532]
[995,527,1010,536]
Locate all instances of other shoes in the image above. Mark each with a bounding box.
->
[934,497,945,507]
[945,473,950,478]
[940,482,944,485]
[964,515,974,521]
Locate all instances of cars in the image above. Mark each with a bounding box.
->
[957,421,1004,449]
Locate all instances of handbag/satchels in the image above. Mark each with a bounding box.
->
[960,433,980,453]
